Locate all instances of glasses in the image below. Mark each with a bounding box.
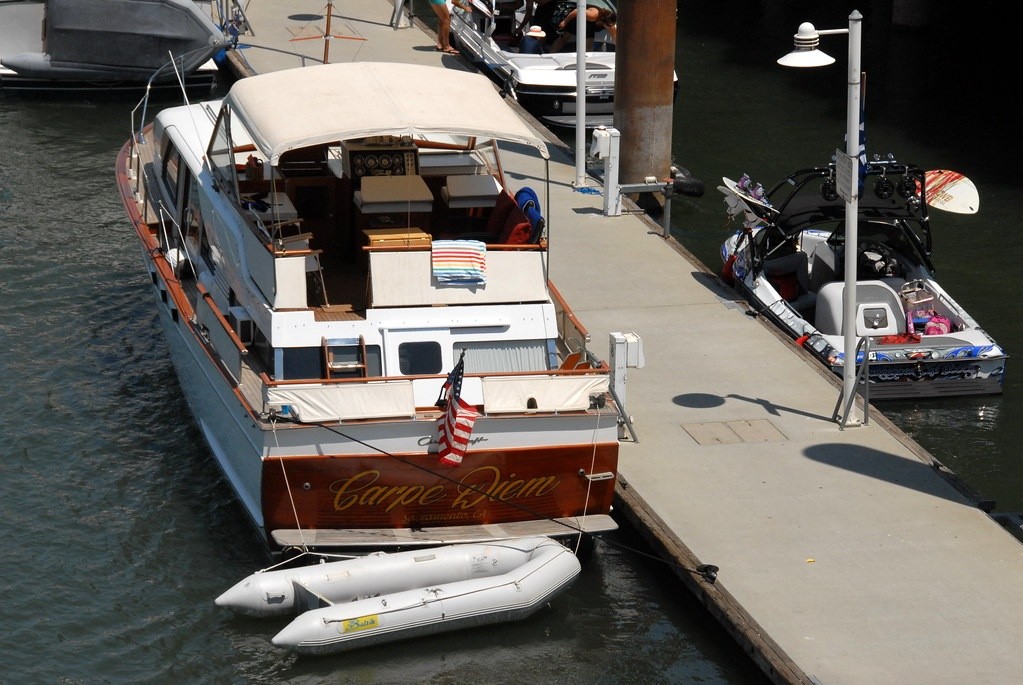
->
[606,23,615,27]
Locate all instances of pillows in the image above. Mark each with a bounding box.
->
[767,269,809,302]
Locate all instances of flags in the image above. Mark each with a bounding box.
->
[428,358,478,469]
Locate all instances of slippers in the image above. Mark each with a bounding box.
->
[437,46,460,54]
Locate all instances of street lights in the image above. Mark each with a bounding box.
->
[776,8,865,428]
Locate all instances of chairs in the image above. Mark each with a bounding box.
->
[322,334,368,384]
[248,203,330,308]
[763,252,816,311]
[438,190,532,244]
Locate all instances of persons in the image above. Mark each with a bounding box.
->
[514,0,617,53]
[519,25,547,54]
[429,0,474,54]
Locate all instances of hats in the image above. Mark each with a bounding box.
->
[525,25,546,37]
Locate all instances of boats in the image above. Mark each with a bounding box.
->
[112,38,641,560]
[0,0,239,96]
[443,0,681,132]
[211,531,582,658]
[715,72,1010,401]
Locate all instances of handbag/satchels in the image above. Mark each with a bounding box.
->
[551,2,577,28]
[925,315,951,336]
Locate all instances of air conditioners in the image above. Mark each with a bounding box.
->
[228,306,253,345]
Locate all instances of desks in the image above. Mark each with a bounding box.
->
[240,192,298,221]
